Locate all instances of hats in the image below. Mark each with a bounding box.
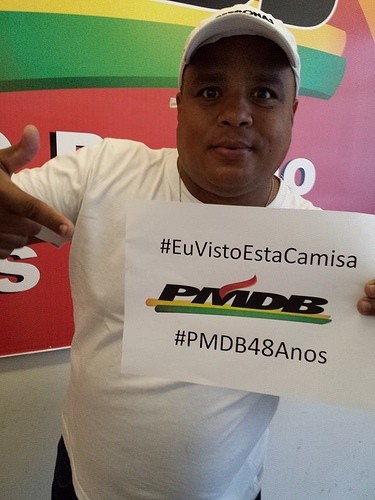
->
[177,3,301,98]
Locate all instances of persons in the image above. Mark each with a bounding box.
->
[0,3,375,500]
